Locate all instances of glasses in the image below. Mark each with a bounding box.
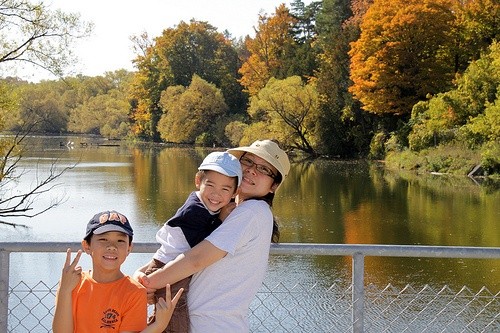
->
[240,156,280,181]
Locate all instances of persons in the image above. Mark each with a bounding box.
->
[132,139,291,333]
[143,151,243,333]
[52,210,184,333]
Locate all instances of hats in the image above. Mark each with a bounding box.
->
[84,211,134,241]
[226,140,290,191]
[198,152,242,187]
